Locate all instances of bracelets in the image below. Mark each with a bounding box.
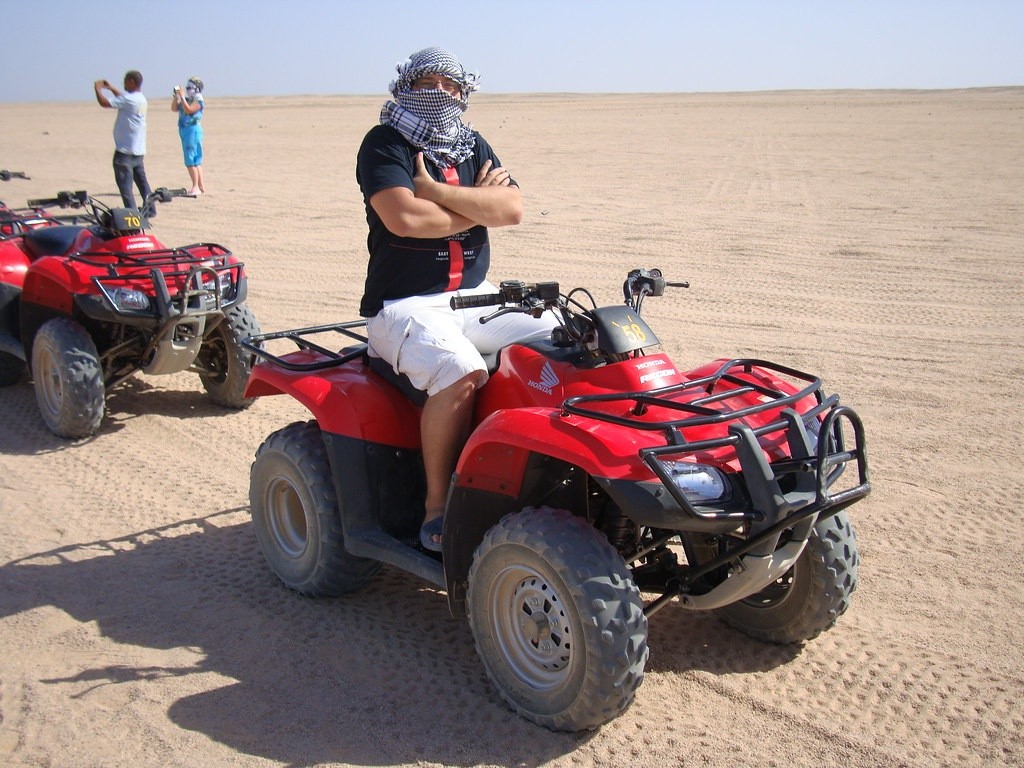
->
[181,97,185,100]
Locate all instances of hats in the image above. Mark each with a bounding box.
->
[190,76,204,92]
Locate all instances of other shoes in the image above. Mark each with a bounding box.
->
[187,189,202,195]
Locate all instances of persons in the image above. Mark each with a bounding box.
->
[95,71,157,217]
[355,47,566,552]
[171,77,206,196]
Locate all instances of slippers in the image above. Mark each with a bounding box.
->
[420,516,443,552]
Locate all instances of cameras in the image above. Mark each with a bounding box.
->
[101,81,106,86]
[174,85,180,94]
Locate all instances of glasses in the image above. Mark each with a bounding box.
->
[186,86,194,89]
[409,80,461,93]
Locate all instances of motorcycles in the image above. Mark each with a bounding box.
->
[241,266,874,735]
[1,170,269,437]
